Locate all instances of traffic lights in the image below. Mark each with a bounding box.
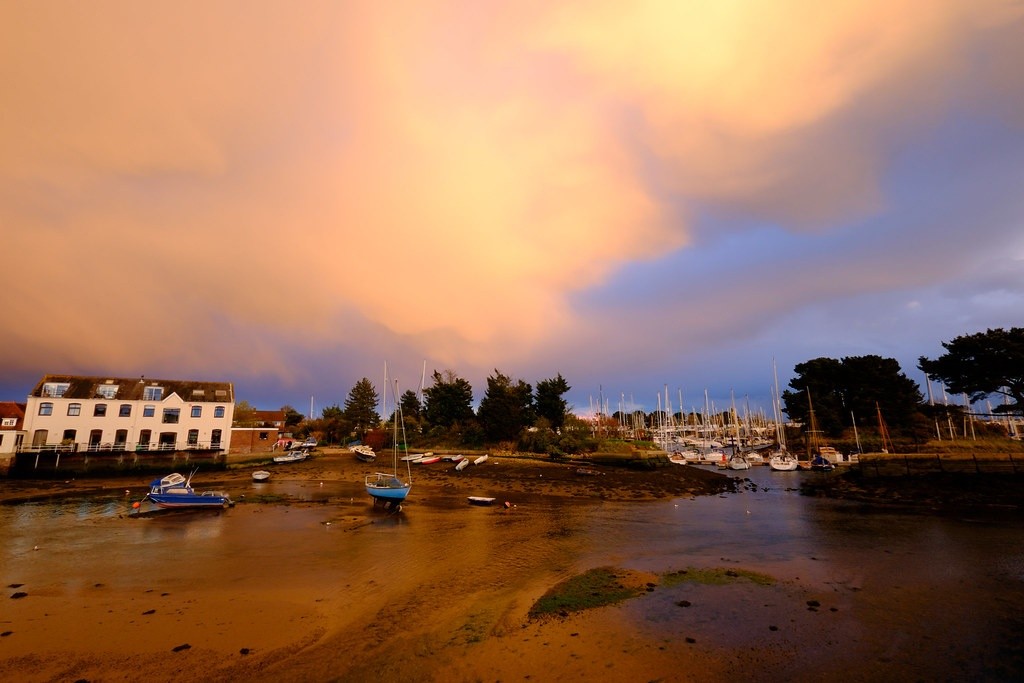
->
[412,456,435,464]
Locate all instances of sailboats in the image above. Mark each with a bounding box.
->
[364,378,411,504]
[589,358,864,470]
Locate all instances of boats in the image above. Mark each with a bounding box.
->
[252,470,270,480]
[451,454,464,463]
[354,444,376,463]
[146,488,230,509]
[467,496,496,505]
[301,437,319,450]
[148,473,193,495]
[474,454,488,465]
[401,454,423,461]
[422,456,441,465]
[455,458,469,470]
[273,450,307,463]
